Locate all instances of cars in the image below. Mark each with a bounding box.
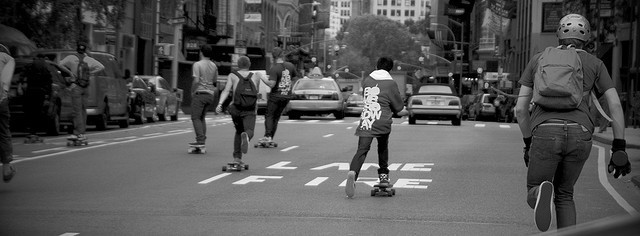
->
[406,82,463,127]
[344,93,364,115]
[127,75,159,125]
[474,94,500,120]
[285,73,345,119]
[131,74,180,122]
[7,57,89,136]
[467,93,483,120]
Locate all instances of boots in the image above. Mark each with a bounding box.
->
[345,171,357,197]
[378,173,389,186]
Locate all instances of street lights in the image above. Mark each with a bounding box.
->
[428,12,464,101]
[283,2,322,56]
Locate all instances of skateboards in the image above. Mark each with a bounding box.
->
[370,181,396,197]
[254,137,277,147]
[221,160,249,172]
[187,140,206,154]
[24,134,43,144]
[66,134,89,147]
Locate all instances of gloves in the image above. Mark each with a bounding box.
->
[608,138,632,179]
[523,136,532,167]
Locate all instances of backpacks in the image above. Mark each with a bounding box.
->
[72,53,90,88]
[530,46,590,110]
[276,62,292,96]
[230,69,257,111]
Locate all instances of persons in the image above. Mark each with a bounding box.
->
[58,36,105,138]
[17,48,53,138]
[515,14,631,232]
[492,94,514,123]
[215,56,260,164]
[0,44,16,183]
[255,46,297,144]
[299,55,324,79]
[345,56,403,197]
[189,45,218,146]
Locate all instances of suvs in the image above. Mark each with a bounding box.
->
[30,48,131,131]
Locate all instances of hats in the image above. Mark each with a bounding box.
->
[238,56,251,68]
[76,42,87,53]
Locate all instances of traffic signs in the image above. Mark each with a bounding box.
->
[167,18,186,26]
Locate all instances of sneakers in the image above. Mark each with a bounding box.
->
[77,134,86,140]
[188,142,205,148]
[3,162,16,183]
[233,159,245,165]
[241,132,249,155]
[533,181,555,232]
[259,137,272,144]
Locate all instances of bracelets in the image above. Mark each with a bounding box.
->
[218,103,223,106]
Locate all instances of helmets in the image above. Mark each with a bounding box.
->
[557,14,591,42]
